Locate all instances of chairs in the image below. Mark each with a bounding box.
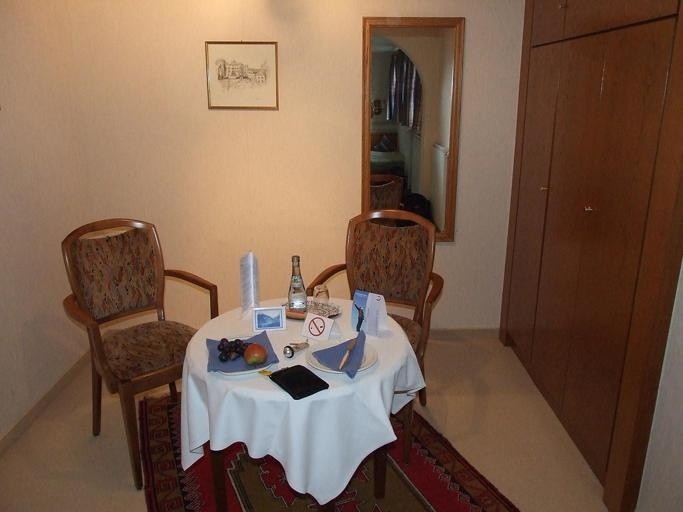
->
[305,207,445,406]
[60,216,219,492]
[369,173,404,227]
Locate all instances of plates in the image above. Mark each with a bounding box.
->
[305,339,379,373]
[282,300,342,318]
[216,336,273,376]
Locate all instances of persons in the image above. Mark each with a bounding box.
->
[354,302,364,331]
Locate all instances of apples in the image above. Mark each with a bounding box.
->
[244,343,267,364]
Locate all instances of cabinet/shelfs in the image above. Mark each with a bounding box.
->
[499,1,682,512]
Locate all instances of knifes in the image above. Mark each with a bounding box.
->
[338,338,356,370]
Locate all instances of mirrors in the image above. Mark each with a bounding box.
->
[362,16,467,244]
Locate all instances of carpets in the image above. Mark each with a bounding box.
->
[137,390,524,512]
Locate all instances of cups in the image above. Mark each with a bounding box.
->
[313,285,330,303]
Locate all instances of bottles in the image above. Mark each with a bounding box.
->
[287,256,307,320]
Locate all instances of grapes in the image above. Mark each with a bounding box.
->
[218,338,246,362]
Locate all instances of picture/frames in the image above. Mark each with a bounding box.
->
[204,40,279,111]
